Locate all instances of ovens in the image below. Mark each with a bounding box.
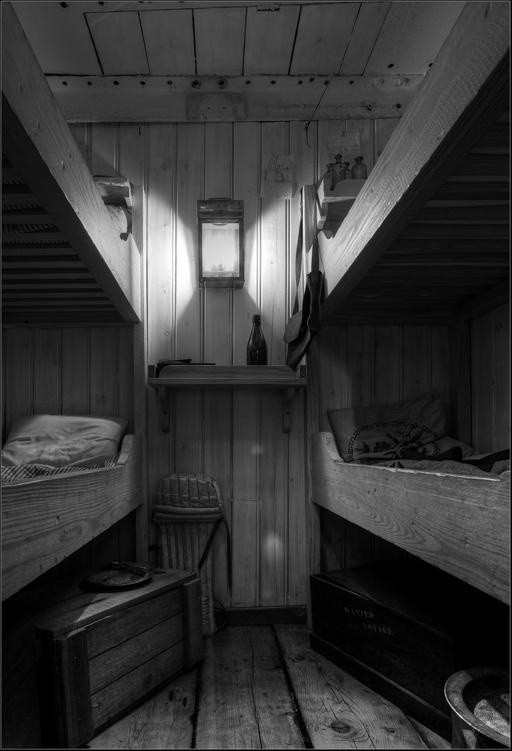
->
[286,1,511,640]
[1,1,147,750]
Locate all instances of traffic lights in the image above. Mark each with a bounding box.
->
[324,392,451,466]
[1,411,128,472]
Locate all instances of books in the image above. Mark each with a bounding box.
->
[2,567,204,749]
[307,560,511,750]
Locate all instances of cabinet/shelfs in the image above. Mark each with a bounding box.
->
[326,153,367,189]
[245,315,267,365]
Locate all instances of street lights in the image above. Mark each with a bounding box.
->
[281,269,328,372]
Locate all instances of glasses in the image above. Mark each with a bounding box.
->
[195,198,246,288]
